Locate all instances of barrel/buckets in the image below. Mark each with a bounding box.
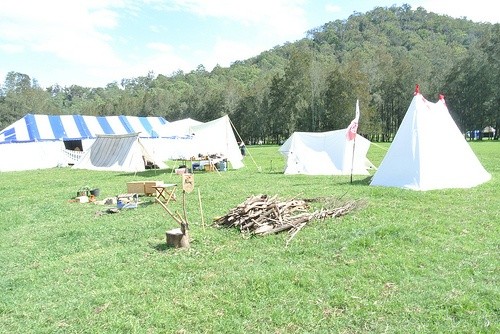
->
[77,191,88,197]
[90,188,100,196]
[219,161,227,171]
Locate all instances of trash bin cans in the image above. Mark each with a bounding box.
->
[241,145,246,157]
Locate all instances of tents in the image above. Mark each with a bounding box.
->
[369,83,491,191]
[0,113,169,172]
[278,128,375,175]
[71,132,169,172]
[152,114,245,169]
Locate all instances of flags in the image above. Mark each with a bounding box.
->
[346,100,360,141]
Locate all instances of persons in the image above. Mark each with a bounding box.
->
[239,141,245,156]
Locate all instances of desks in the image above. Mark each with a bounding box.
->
[171,156,226,178]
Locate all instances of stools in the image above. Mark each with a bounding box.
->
[152,184,178,205]
